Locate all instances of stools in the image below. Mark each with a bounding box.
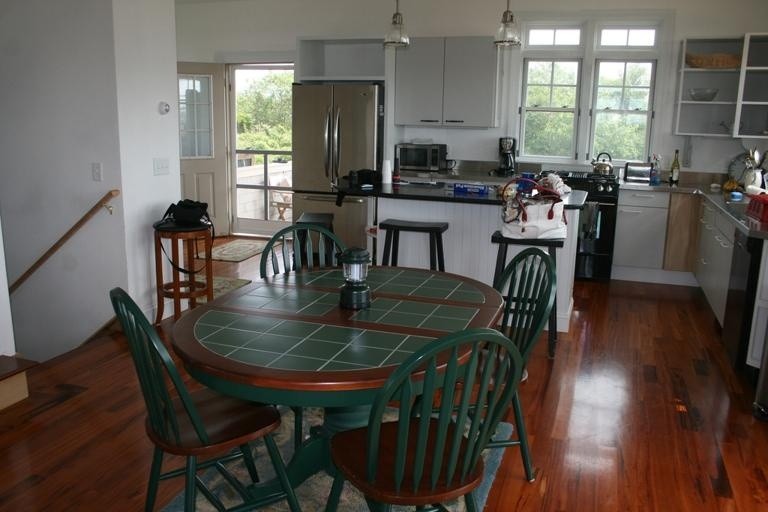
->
[492,230,563,359]
[292,211,335,270]
[152,219,214,328]
[379,218,448,273]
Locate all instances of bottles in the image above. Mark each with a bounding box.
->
[669,149,679,183]
[650,158,661,186]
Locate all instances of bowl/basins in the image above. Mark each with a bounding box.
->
[689,88,717,100]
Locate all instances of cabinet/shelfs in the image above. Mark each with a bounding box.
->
[692,198,748,352]
[291,34,386,81]
[610,190,670,289]
[394,36,503,129]
[663,193,702,272]
[673,32,768,139]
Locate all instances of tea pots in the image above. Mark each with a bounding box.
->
[592,154,614,174]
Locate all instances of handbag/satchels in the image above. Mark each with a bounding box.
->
[336,169,381,206]
[501,178,567,239]
[172,199,208,226]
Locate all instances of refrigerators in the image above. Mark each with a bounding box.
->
[292,81,377,258]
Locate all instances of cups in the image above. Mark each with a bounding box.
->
[744,168,763,189]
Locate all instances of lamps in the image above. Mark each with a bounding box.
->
[383,0,410,48]
[494,0,522,46]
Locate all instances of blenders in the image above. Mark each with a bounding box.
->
[497,138,515,176]
[336,246,373,310]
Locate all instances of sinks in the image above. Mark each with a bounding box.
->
[726,201,750,214]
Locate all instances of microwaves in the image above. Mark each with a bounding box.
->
[396,143,448,172]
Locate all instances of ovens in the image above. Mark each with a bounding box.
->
[576,195,618,280]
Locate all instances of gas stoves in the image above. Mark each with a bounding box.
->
[538,171,616,195]
